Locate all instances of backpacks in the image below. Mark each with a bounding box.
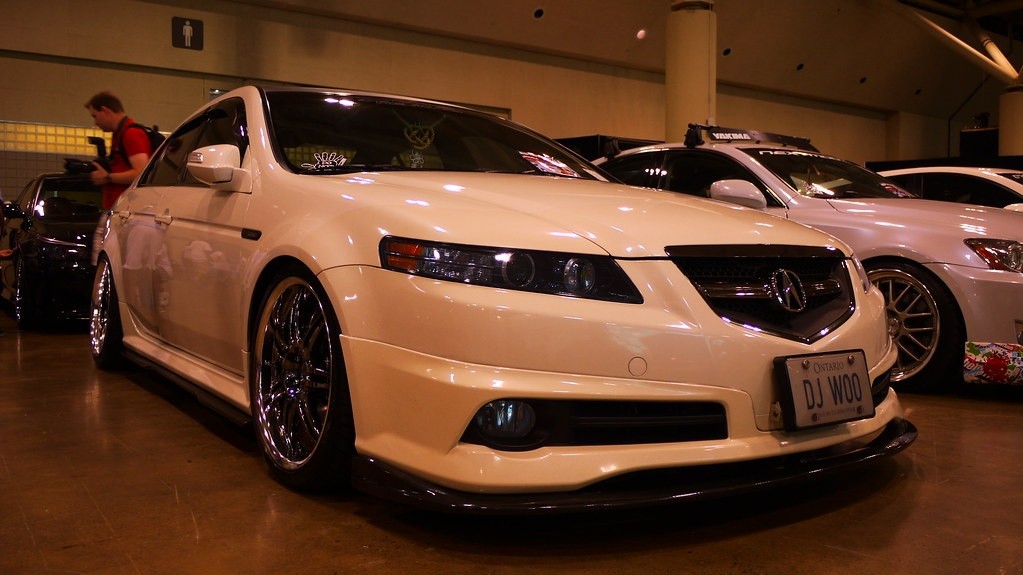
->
[120,122,165,169]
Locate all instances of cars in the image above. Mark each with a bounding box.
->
[817,166,1023,212]
[590,122,1022,396]
[90,84,919,520]
[0,172,102,334]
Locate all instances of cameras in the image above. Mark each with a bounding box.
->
[61,136,110,174]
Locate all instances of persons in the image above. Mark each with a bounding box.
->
[84,91,152,266]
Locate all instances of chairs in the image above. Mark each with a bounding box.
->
[43,197,77,217]
[349,138,394,164]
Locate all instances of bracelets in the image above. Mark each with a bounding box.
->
[107,174,112,182]
